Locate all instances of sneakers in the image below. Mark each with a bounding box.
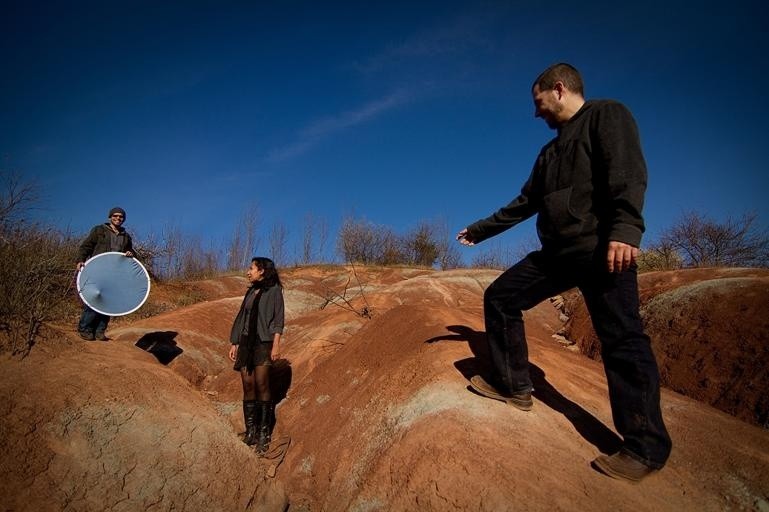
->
[79,331,94,340]
[470,374,533,411]
[95,332,107,341]
[593,451,655,483]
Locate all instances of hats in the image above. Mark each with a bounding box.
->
[108,208,126,221]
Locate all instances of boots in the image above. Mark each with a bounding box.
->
[254,400,271,453]
[242,399,256,446]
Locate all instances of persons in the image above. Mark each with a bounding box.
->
[227,257,285,455]
[75,206,137,342]
[454,62,672,484]
